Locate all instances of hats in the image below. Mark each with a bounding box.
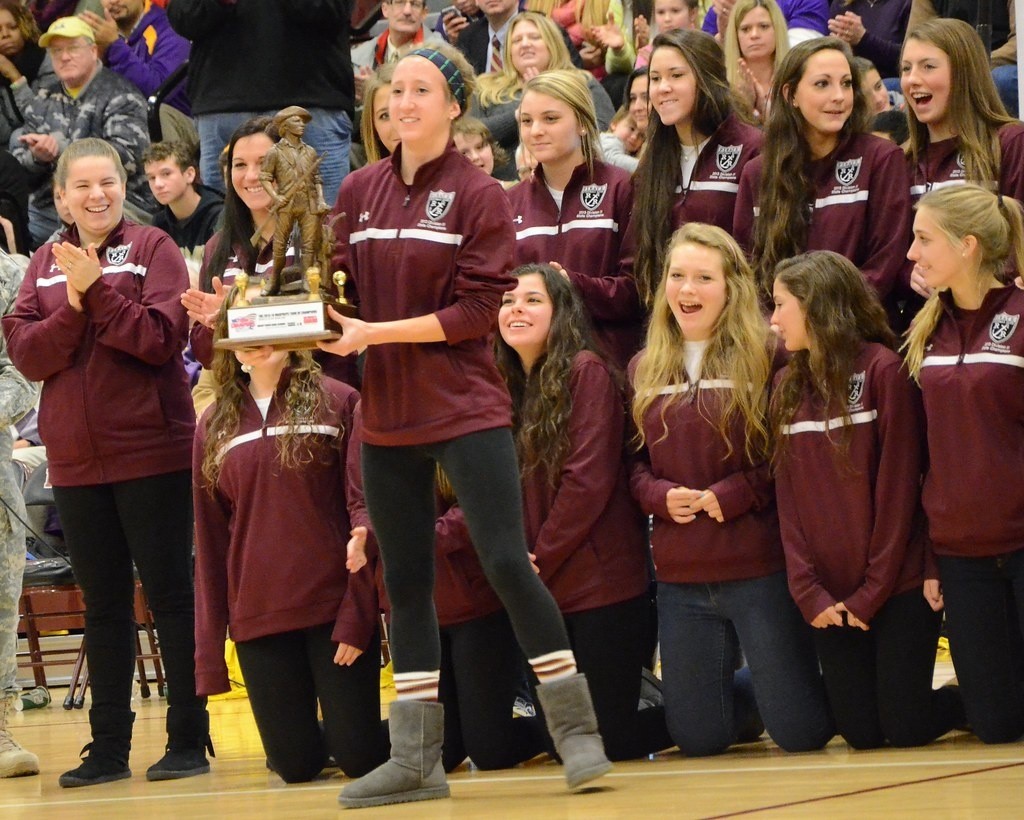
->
[38,16,96,43]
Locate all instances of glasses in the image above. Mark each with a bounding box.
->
[46,45,86,58]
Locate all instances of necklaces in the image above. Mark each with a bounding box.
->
[680,142,699,162]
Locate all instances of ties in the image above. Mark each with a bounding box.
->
[491,34,503,73]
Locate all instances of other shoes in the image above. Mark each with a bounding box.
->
[638,663,665,709]
[512,696,537,718]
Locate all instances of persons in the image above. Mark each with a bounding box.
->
[731,38,913,303]
[0,0,1024,559]
[632,28,769,325]
[771,254,966,750]
[900,16,1024,300]
[192,277,390,781]
[181,43,616,803]
[622,224,839,754]
[502,67,634,325]
[1,243,42,776]
[905,185,1024,746]
[261,105,323,296]
[346,339,549,771]
[2,139,215,787]
[487,264,763,768]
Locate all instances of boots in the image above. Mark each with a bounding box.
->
[57,710,136,789]
[1,692,39,778]
[146,706,215,780]
[336,698,450,808]
[535,670,613,787]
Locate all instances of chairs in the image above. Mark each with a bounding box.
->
[19,461,166,710]
